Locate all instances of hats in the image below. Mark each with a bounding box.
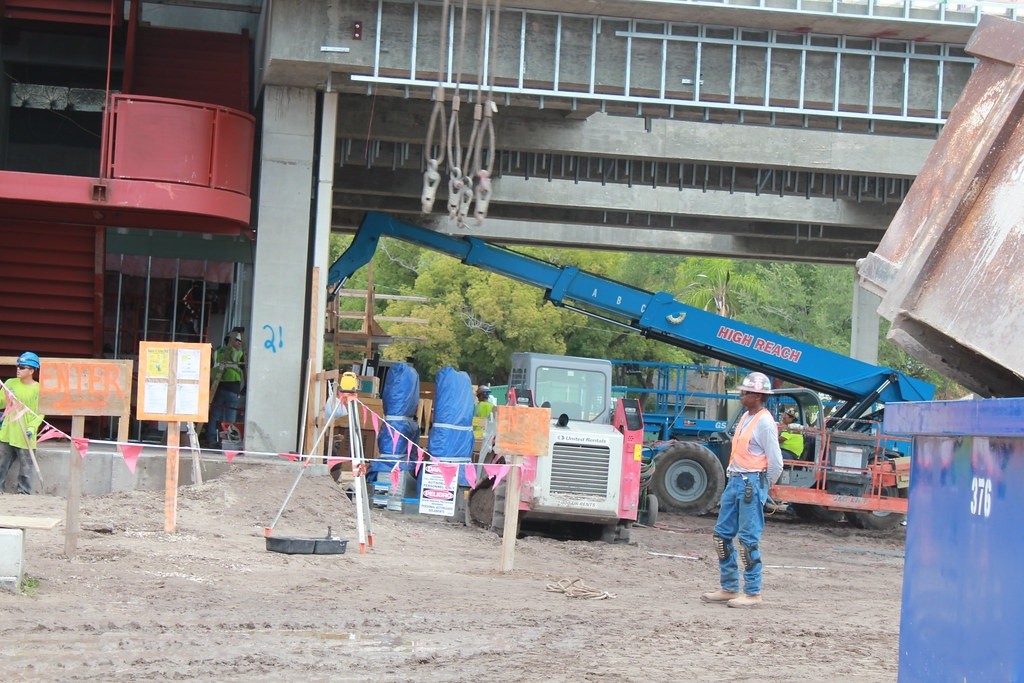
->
[785,408,798,420]
[17,352,40,368]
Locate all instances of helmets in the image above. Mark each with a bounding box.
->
[735,372,775,395]
[476,385,492,397]
[226,331,243,342]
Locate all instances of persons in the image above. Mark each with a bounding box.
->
[472,385,498,432]
[0,352,46,495]
[700,371,785,609]
[768,406,805,470]
[208,330,249,450]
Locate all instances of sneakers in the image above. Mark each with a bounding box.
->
[726,593,762,610]
[701,591,738,604]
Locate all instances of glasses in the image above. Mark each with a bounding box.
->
[18,364,28,370]
[740,390,759,396]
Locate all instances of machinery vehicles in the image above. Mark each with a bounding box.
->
[327,206,938,537]
